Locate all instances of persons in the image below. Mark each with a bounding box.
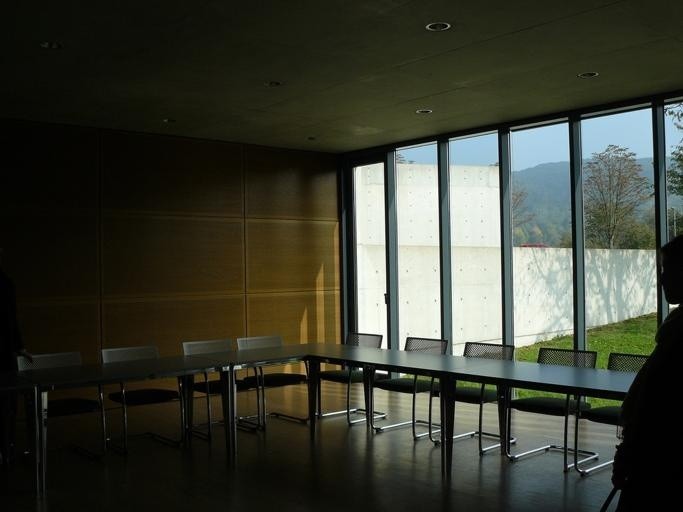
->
[0,270,35,451]
[610,233,683,510]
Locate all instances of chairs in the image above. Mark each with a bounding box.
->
[17,350,107,468]
[183,337,260,441]
[235,335,312,436]
[368,336,449,440]
[99,344,187,455]
[429,341,516,453]
[505,347,599,474]
[320,332,386,426]
[575,352,649,476]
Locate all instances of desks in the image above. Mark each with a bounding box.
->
[9,341,315,454]
[316,343,640,456]
[0,360,40,500]
